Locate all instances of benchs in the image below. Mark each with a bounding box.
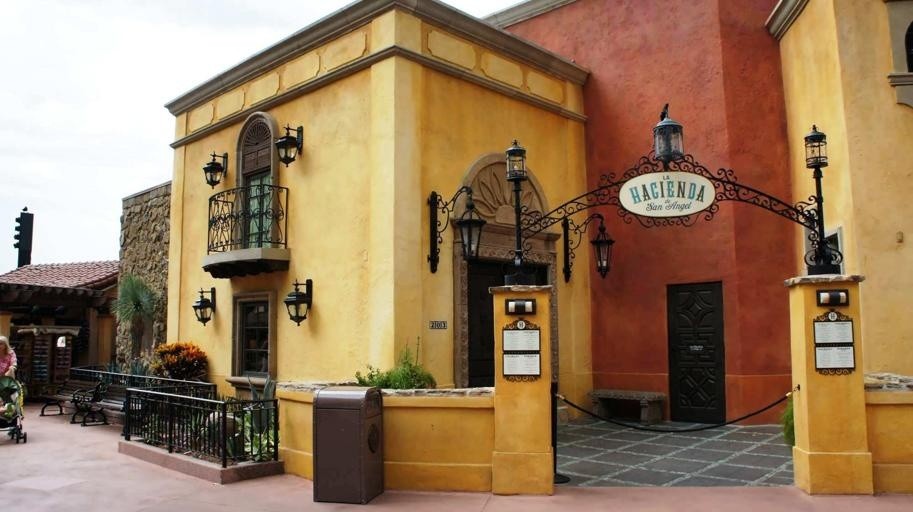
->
[37,379,141,427]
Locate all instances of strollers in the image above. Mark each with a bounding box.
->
[0,375,28,444]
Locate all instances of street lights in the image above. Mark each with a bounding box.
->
[505,139,536,284]
[804,123,842,275]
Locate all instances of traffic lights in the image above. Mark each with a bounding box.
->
[14,212,33,248]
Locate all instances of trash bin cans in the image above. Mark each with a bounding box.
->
[313,386,384,505]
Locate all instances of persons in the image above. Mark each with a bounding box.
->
[0,336,18,378]
[0,397,12,421]
